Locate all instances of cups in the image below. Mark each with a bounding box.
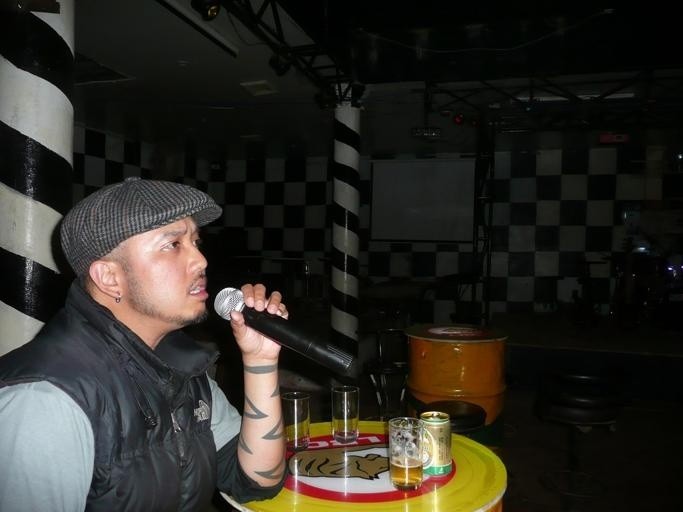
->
[331,386,359,444]
[281,392,310,452]
[388,417,424,491]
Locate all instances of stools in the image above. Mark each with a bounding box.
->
[532,386,622,512]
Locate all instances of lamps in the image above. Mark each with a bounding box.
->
[189,0,371,112]
[411,75,655,132]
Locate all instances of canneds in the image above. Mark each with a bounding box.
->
[419,411,453,476]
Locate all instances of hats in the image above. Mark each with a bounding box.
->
[59,176,223,277]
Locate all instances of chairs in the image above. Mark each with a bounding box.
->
[366,330,408,417]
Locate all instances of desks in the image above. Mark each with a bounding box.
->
[399,323,509,428]
[217,418,507,512]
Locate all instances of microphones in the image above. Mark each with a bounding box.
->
[215,288,354,375]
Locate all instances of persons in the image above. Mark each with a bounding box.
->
[0,176,289,512]
